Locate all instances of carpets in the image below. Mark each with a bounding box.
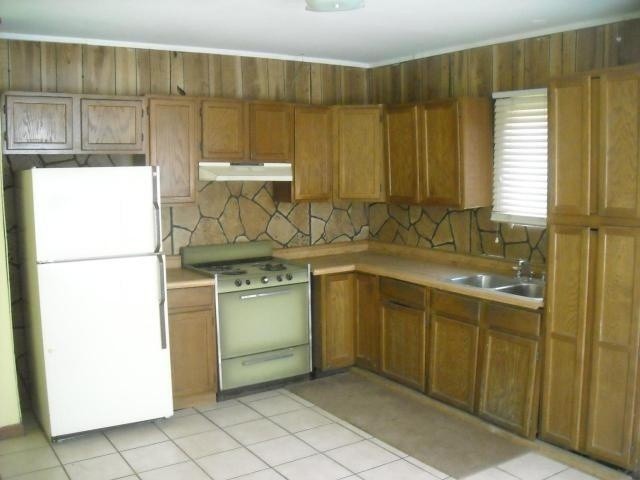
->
[282,369,536,480]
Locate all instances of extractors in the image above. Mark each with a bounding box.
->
[198,158,294,182]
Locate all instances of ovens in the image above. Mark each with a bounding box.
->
[214,281,316,401]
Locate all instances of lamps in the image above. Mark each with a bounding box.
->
[305,0,365,12]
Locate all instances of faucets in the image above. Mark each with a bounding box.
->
[518,259,538,278]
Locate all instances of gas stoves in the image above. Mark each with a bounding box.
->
[184,256,311,294]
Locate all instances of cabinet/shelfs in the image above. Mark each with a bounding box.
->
[1,90,147,158]
[380,273,431,394]
[273,101,334,206]
[355,266,380,376]
[310,266,357,380]
[198,95,295,165]
[426,281,483,416]
[383,95,495,212]
[148,92,199,207]
[334,103,389,204]
[546,60,639,219]
[167,280,219,408]
[538,218,639,478]
[474,292,546,442]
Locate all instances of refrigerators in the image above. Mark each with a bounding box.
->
[12,165,175,444]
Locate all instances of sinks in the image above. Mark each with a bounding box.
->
[439,271,519,290]
[490,280,547,303]
